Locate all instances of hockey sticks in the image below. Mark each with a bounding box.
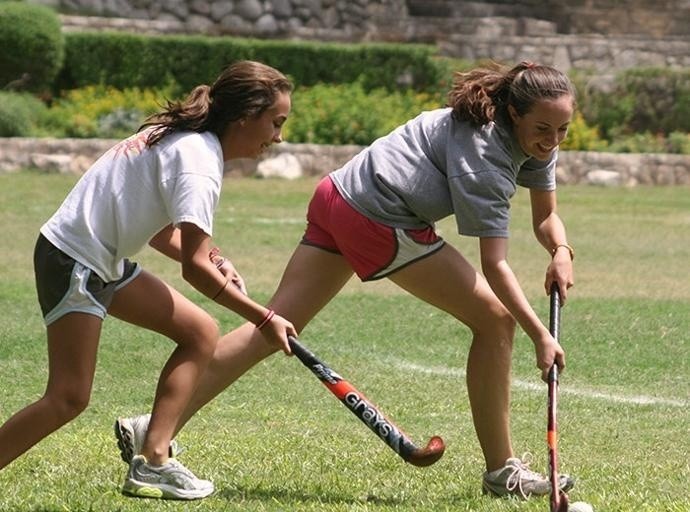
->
[548,283,569,512]
[286,335,445,466]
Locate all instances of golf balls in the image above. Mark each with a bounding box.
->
[567,500,594,512]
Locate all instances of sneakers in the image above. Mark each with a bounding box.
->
[115,414,215,500]
[482,458,573,498]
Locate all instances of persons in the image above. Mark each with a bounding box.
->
[113,60,581,500]
[0,61,300,505]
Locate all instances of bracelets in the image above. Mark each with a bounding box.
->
[550,243,575,262]
[255,309,275,332]
[210,279,233,300]
